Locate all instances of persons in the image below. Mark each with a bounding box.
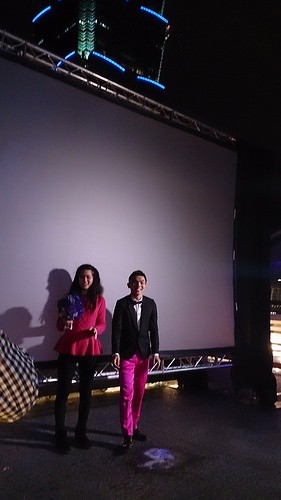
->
[53,264,107,454]
[0,327,39,423]
[111,269,161,447]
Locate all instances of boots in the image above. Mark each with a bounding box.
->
[75,425,90,449]
[55,436,69,455]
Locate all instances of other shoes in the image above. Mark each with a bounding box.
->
[133,429,148,441]
[122,436,137,448]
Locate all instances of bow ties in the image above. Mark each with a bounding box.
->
[133,301,143,305]
